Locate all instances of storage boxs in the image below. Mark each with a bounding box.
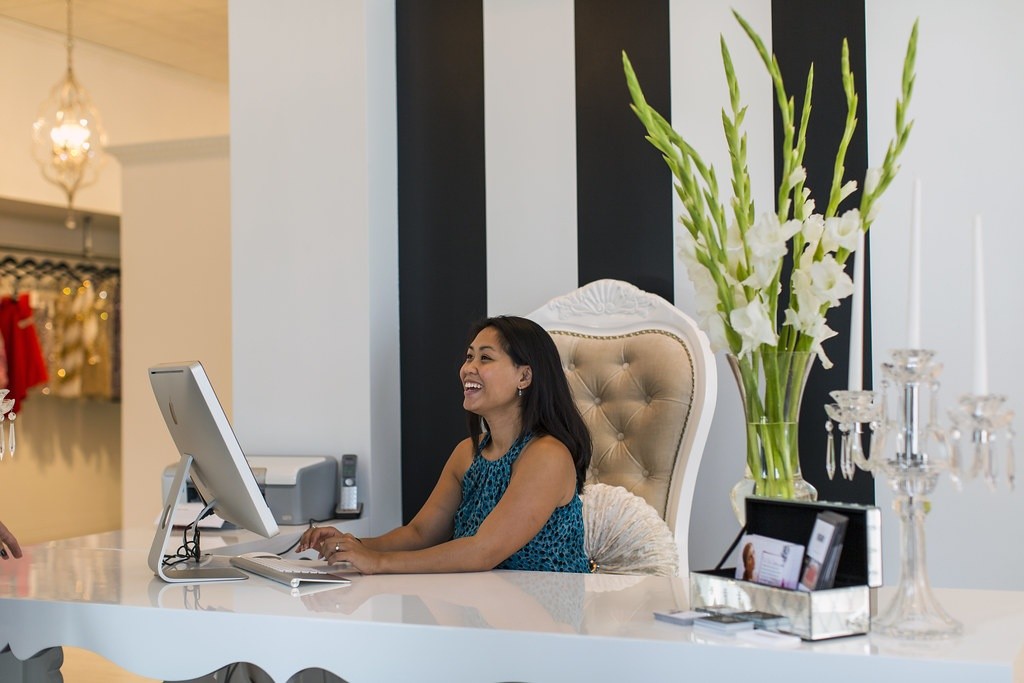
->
[688,494,884,642]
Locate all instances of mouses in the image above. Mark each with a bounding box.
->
[238,552,281,559]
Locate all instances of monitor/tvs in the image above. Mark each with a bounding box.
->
[148,360,279,537]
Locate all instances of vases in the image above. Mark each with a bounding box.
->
[728,349,819,531]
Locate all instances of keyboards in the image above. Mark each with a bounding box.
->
[230,557,352,588]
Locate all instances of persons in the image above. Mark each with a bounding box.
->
[742,543,758,583]
[295,314,594,574]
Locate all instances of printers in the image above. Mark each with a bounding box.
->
[161,454,340,525]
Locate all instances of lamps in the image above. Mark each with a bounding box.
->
[825,348,1018,641]
[30,1,106,231]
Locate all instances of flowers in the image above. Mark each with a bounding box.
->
[620,7,919,370]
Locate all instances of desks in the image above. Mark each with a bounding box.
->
[0,512,1024,683]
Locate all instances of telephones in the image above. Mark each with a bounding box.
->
[335,454,364,518]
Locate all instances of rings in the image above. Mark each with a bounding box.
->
[312,525,317,530]
[335,542,339,552]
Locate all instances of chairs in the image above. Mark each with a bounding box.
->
[519,277,718,579]
